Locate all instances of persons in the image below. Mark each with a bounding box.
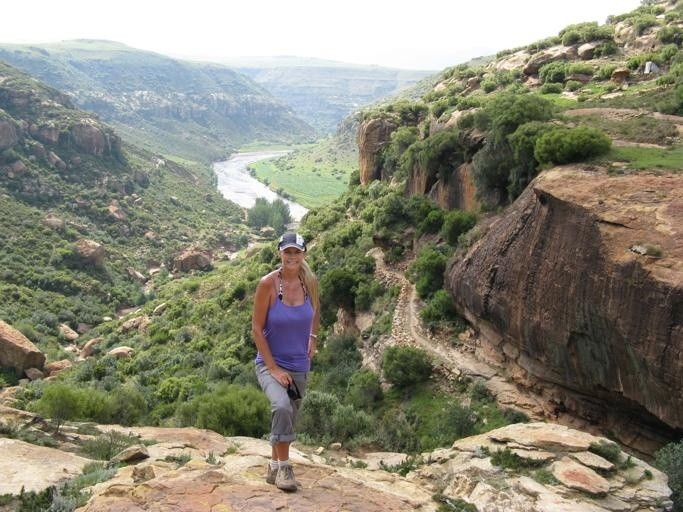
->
[252,233,320,491]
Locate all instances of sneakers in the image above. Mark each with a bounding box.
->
[266,463,301,491]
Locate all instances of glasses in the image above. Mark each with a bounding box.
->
[287,379,302,401]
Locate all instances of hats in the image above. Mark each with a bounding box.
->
[278,233,307,252]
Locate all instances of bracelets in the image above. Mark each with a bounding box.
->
[310,334,318,338]
